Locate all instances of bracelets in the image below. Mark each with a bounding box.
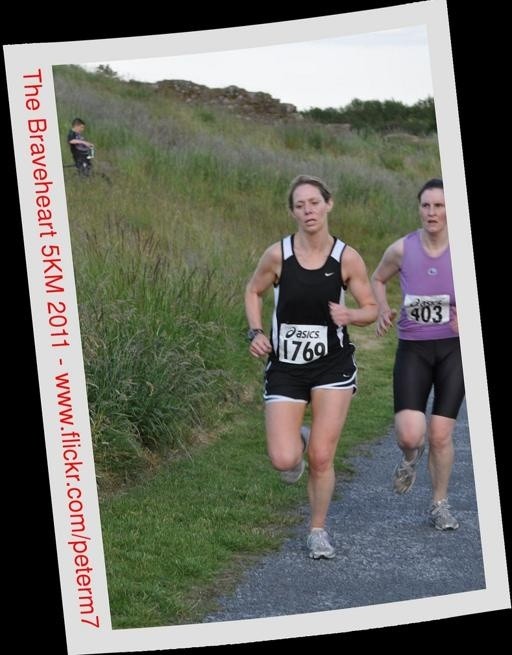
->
[246,327,267,340]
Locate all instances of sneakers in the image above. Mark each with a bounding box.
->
[390,444,425,494]
[429,499,458,530]
[307,531,335,559]
[280,426,311,483]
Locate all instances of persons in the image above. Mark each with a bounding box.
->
[370,178,472,532]
[244,174,380,560]
[66,117,96,175]
